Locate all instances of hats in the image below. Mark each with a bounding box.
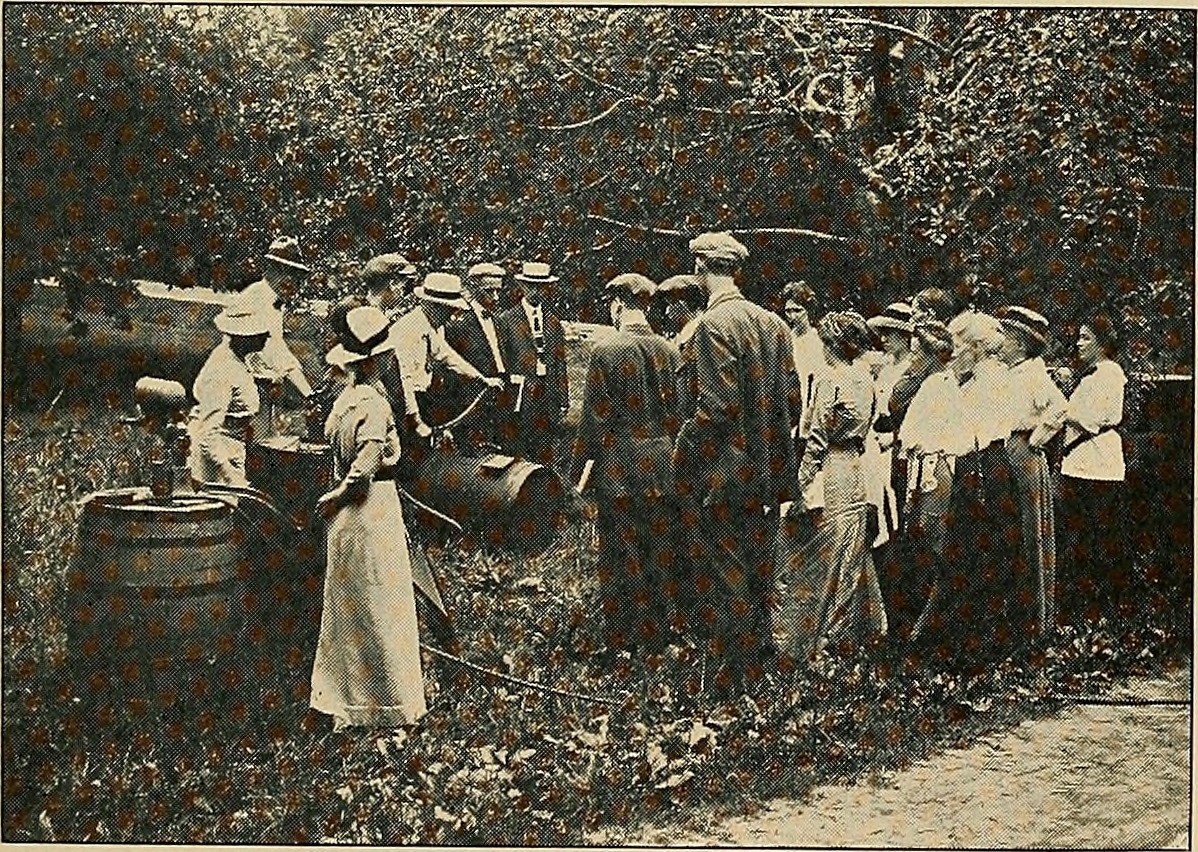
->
[513,262,562,282]
[657,274,708,299]
[364,253,419,279]
[214,298,273,335]
[689,233,748,263]
[601,272,659,300]
[1001,305,1052,350]
[469,263,506,277]
[264,236,310,272]
[413,273,472,311]
[866,303,916,336]
[324,308,405,365]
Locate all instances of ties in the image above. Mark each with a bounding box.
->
[533,310,544,350]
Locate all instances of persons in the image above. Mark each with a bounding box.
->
[186,233,1127,729]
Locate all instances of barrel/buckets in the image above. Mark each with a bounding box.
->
[68,487,240,726]
[413,450,563,558]
[253,436,334,530]
